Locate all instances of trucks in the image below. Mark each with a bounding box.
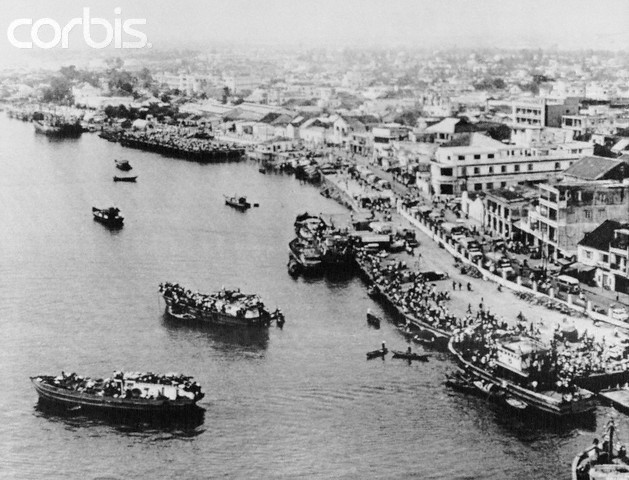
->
[460,237,483,262]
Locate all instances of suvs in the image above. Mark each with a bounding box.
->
[442,223,466,244]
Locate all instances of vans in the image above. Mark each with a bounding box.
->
[556,275,580,293]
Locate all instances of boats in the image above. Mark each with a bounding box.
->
[392,350,432,358]
[93,207,124,226]
[113,175,137,181]
[447,327,597,416]
[158,282,270,326]
[295,212,352,264]
[226,197,250,210]
[30,370,204,413]
[572,417,629,480]
[367,348,386,358]
[33,119,61,134]
[367,309,378,327]
[289,237,322,268]
[115,159,132,171]
